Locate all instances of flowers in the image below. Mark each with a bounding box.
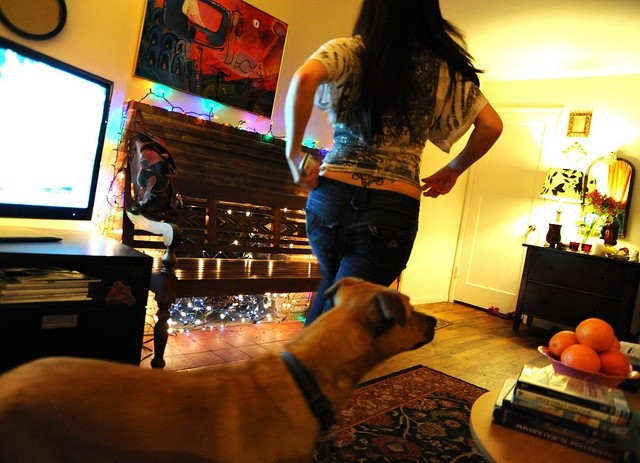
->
[586,189,630,222]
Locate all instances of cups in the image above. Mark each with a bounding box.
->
[569,241,580,253]
[545,223,562,248]
[581,244,592,254]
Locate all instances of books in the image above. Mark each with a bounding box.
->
[1,266,102,307]
[487,362,639,462]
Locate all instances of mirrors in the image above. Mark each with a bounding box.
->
[582,153,639,238]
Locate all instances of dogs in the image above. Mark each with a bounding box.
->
[0,277,436,463]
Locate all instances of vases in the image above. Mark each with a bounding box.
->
[600,218,620,247]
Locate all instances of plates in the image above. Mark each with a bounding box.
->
[537,344,633,390]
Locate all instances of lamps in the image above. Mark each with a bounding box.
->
[539,166,585,246]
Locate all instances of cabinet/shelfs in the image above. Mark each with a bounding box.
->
[513,242,639,340]
[1,226,154,374]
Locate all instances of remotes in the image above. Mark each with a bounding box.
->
[298,154,319,176]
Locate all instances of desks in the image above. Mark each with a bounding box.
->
[470,389,638,463]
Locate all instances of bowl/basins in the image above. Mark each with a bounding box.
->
[618,340,640,367]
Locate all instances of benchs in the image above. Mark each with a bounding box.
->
[121,101,327,369]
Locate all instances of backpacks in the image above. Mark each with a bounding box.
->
[107,108,185,221]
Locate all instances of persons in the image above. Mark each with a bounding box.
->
[284,0,503,330]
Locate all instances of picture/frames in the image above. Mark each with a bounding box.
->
[567,112,592,137]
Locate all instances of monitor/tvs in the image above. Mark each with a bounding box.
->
[0,35,115,242]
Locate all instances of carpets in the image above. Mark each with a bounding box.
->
[311,363,492,462]
